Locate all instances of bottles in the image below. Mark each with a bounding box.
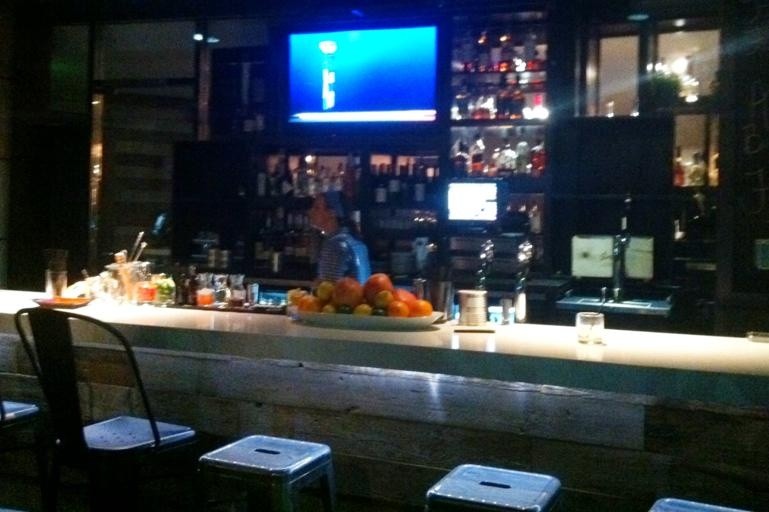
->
[248,282,259,304]
[218,247,229,267]
[228,273,246,306]
[252,206,319,272]
[208,244,217,267]
[411,278,426,301]
[453,25,547,177]
[195,272,216,306]
[214,274,230,305]
[253,153,429,204]
[674,144,720,187]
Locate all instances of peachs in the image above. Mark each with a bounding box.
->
[290,287,307,306]
[298,295,321,313]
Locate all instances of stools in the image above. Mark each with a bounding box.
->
[642,493,739,510]
[420,456,558,512]
[193,431,345,510]
[3,389,53,512]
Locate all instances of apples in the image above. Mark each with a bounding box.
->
[331,278,364,308]
[364,273,393,303]
[394,288,417,306]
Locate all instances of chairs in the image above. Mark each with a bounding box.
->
[9,294,203,511]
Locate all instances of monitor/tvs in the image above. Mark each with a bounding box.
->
[269,22,451,148]
[445,177,508,234]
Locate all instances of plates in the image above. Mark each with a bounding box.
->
[32,297,97,309]
[286,306,444,330]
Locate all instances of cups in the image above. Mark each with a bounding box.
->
[457,289,488,325]
[44,269,68,299]
[136,282,158,302]
[575,311,605,344]
[430,279,451,322]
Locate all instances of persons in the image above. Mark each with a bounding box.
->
[309,190,372,289]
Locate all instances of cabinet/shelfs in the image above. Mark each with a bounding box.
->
[231,141,449,280]
[444,4,557,275]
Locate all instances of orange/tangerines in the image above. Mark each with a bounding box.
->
[322,291,393,316]
[316,282,333,301]
